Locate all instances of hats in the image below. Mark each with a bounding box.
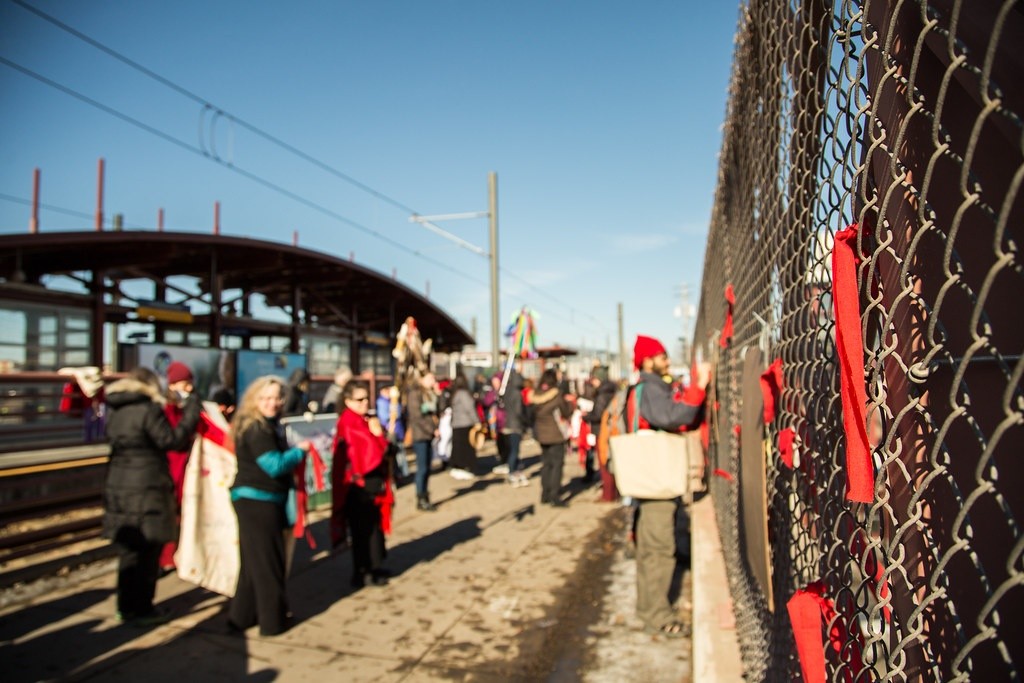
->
[634,334,666,368]
[590,365,611,385]
[168,360,193,384]
[494,372,504,381]
[468,427,485,450]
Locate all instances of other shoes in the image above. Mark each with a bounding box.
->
[129,603,174,626]
[416,498,435,510]
[494,465,510,473]
[659,619,692,638]
[541,497,566,508]
[508,473,530,489]
[450,468,474,479]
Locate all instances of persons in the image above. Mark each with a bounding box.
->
[225,375,313,639]
[402,371,440,512]
[437,365,715,508]
[284,370,311,417]
[157,361,209,575]
[608,334,712,640]
[376,379,406,487]
[321,365,352,410]
[329,377,396,590]
[97,362,235,626]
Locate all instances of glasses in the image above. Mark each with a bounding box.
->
[349,397,368,402]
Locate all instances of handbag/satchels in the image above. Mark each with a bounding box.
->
[609,384,705,500]
[553,407,574,440]
